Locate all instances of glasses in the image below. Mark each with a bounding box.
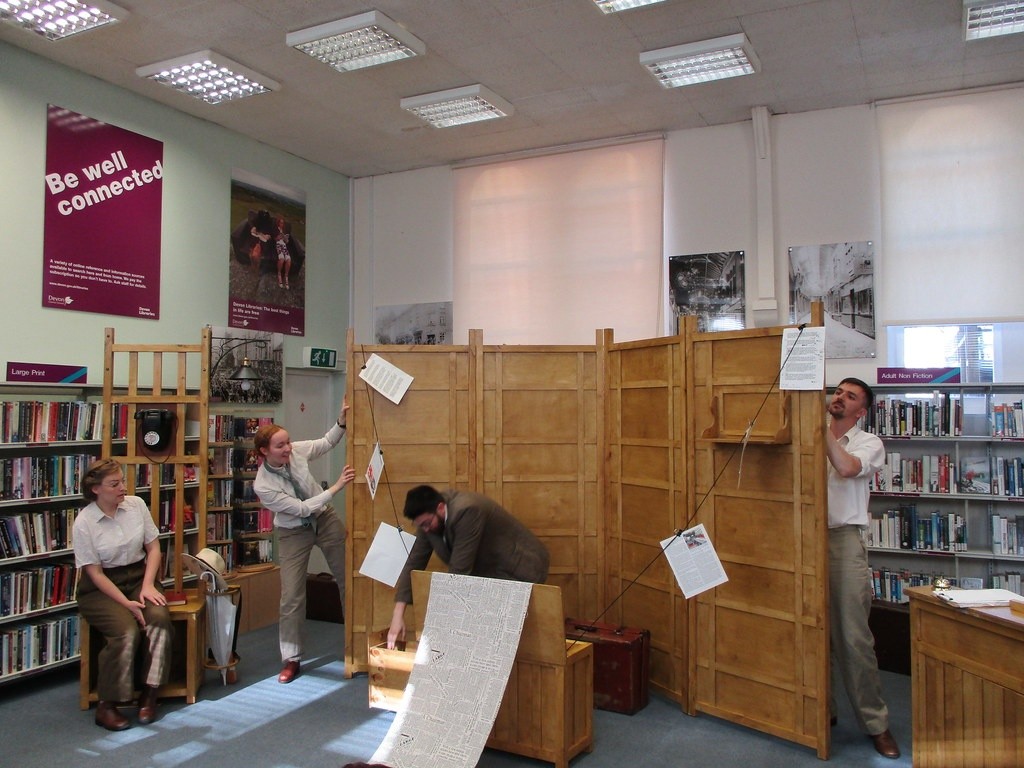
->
[411,512,437,528]
[100,479,126,488]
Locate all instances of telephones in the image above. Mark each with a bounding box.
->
[134,408,176,452]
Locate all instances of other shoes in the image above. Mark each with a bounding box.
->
[276,278,284,289]
[285,283,290,290]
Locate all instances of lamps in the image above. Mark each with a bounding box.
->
[285,10,426,74]
[399,83,513,130]
[135,49,281,107]
[0,0,131,43]
[961,0,1024,43]
[639,32,763,90]
[210,337,263,390]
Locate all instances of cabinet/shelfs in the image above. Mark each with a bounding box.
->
[80,325,212,710]
[826,386,1024,676]
[0,383,281,688]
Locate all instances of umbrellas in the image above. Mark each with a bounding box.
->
[200,572,238,687]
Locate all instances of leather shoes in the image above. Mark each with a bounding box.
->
[95,704,130,731]
[278,660,300,683]
[873,729,900,759]
[137,693,158,724]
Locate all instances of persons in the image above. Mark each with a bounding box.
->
[72,457,175,730]
[387,486,551,649]
[825,378,902,759]
[249,211,292,290]
[252,392,356,684]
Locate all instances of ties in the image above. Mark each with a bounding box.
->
[263,460,317,534]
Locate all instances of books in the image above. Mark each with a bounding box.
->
[826,398,1024,604]
[1,399,273,679]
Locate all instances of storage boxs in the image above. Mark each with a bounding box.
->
[368,639,419,712]
[410,570,594,768]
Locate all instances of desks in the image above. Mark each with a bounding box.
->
[902,587,1024,768]
